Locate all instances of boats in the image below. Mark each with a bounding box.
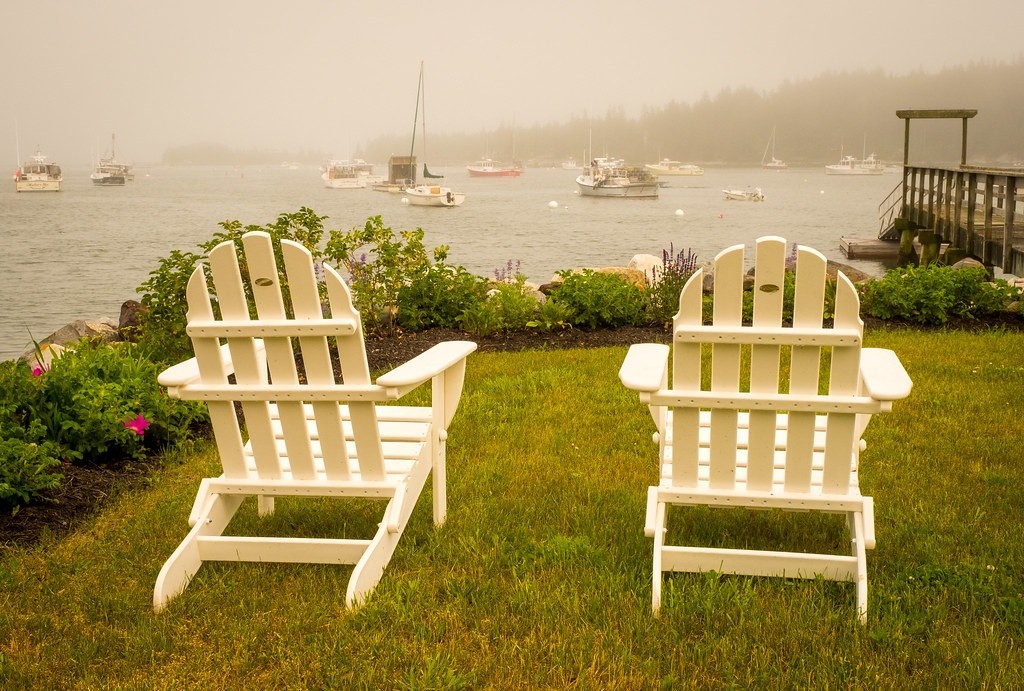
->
[91,129,134,185]
[466,159,523,177]
[724,185,767,202]
[14,125,62,194]
[563,128,707,197]
[826,135,885,176]
[324,161,377,188]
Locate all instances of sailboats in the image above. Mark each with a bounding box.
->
[372,60,464,205]
[761,130,787,169]
